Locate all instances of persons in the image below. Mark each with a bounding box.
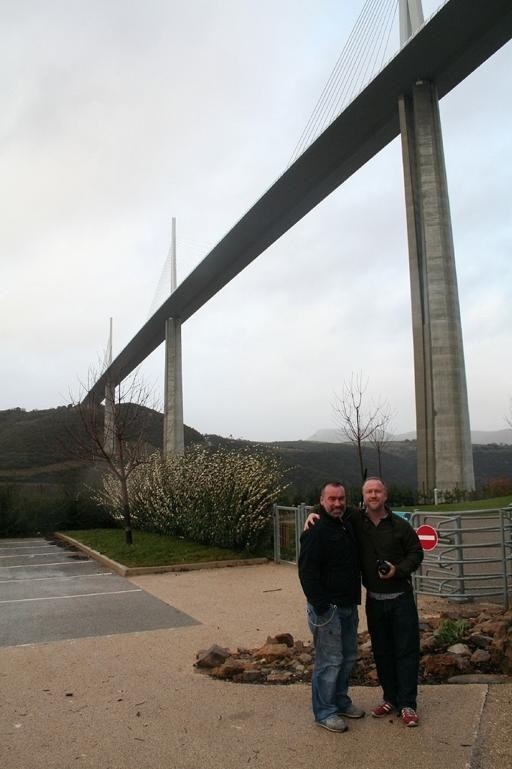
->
[303,475,426,727]
[297,481,367,732]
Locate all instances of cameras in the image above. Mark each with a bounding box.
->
[376,560,391,575]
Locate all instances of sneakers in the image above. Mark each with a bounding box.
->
[337,702,365,718]
[370,701,393,718]
[315,713,346,732]
[401,707,418,727]
